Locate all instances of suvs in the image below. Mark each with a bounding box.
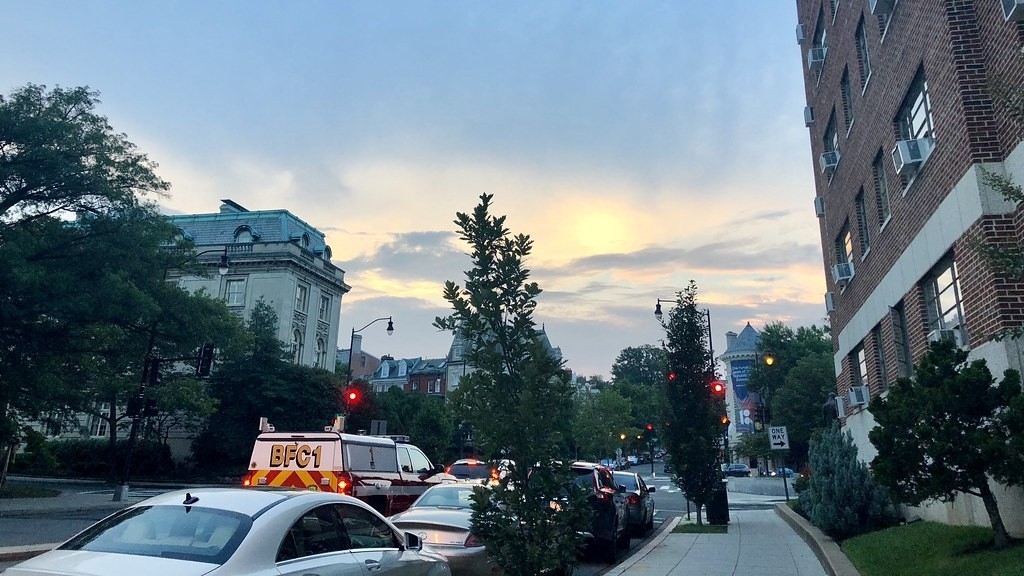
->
[524,458,632,564]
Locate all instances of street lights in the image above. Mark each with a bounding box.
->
[754,352,776,431]
[346,316,395,434]
[112,246,232,502]
[654,298,715,381]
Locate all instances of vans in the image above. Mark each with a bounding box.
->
[239,413,446,517]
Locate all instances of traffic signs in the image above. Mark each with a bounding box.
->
[768,426,790,450]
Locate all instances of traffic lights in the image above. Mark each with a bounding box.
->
[348,385,360,413]
[721,412,728,427]
[196,340,214,379]
[646,420,653,434]
[714,382,723,399]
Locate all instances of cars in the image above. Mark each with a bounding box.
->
[597,448,674,474]
[0,484,453,576]
[373,482,526,576]
[757,467,796,478]
[609,470,656,539]
[351,458,517,529]
[720,463,752,478]
